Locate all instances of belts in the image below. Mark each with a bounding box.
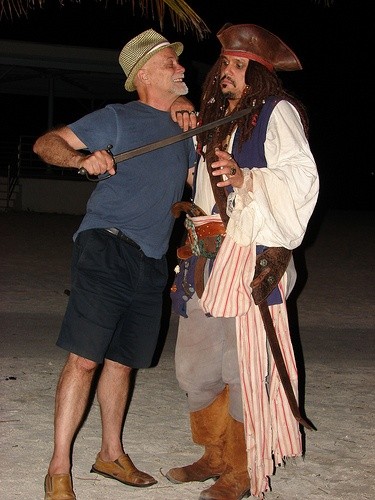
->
[194,221,226,240]
[107,228,140,249]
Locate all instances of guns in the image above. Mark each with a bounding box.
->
[170,201,207,218]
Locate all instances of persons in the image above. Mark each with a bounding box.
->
[165,22,320,500]
[33,28,197,500]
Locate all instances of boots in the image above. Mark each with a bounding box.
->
[166,384,229,483]
[200,415,251,500]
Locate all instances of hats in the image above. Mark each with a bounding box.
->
[119,28,184,92]
[216,22,302,72]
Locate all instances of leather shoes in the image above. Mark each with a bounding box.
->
[90,453,157,488]
[44,472,76,500]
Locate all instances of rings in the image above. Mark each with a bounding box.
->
[229,167,236,176]
[190,111,196,115]
[176,111,182,114]
[182,110,189,114]
[228,154,234,161]
